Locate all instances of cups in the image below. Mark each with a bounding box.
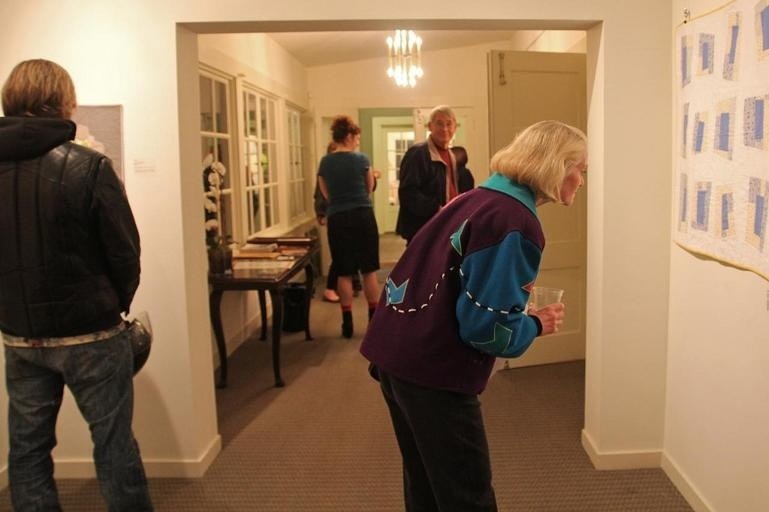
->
[532,286,564,311]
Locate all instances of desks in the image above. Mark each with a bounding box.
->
[209,237,317,389]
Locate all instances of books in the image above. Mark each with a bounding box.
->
[233,232,317,260]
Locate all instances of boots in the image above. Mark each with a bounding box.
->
[342,311,353,337]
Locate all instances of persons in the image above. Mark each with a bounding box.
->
[0,56,157,511]
[317,111,381,340]
[360,119,587,512]
[394,104,459,250]
[448,146,475,196]
[314,141,377,303]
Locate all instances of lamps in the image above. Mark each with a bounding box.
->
[386,29,425,90]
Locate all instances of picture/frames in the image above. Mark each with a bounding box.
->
[76,102,124,189]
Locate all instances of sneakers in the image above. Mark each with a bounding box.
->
[324,290,340,302]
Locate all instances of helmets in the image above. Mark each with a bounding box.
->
[124,311,152,376]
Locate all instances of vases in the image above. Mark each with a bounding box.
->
[208,235,225,275]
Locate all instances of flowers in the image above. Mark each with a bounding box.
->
[201,153,226,236]
